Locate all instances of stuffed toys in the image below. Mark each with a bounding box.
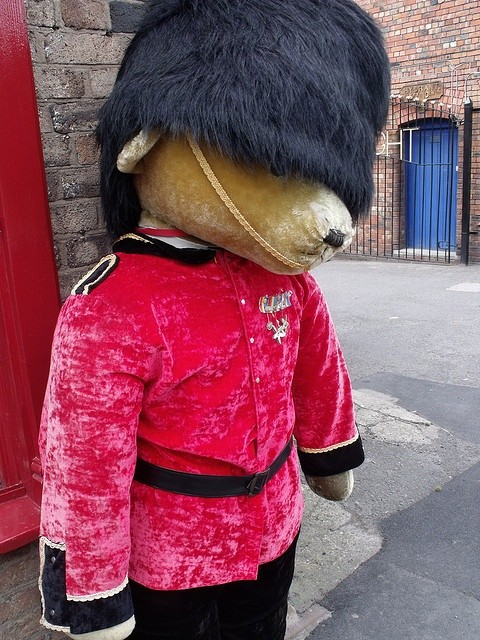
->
[39,1,393,636]
